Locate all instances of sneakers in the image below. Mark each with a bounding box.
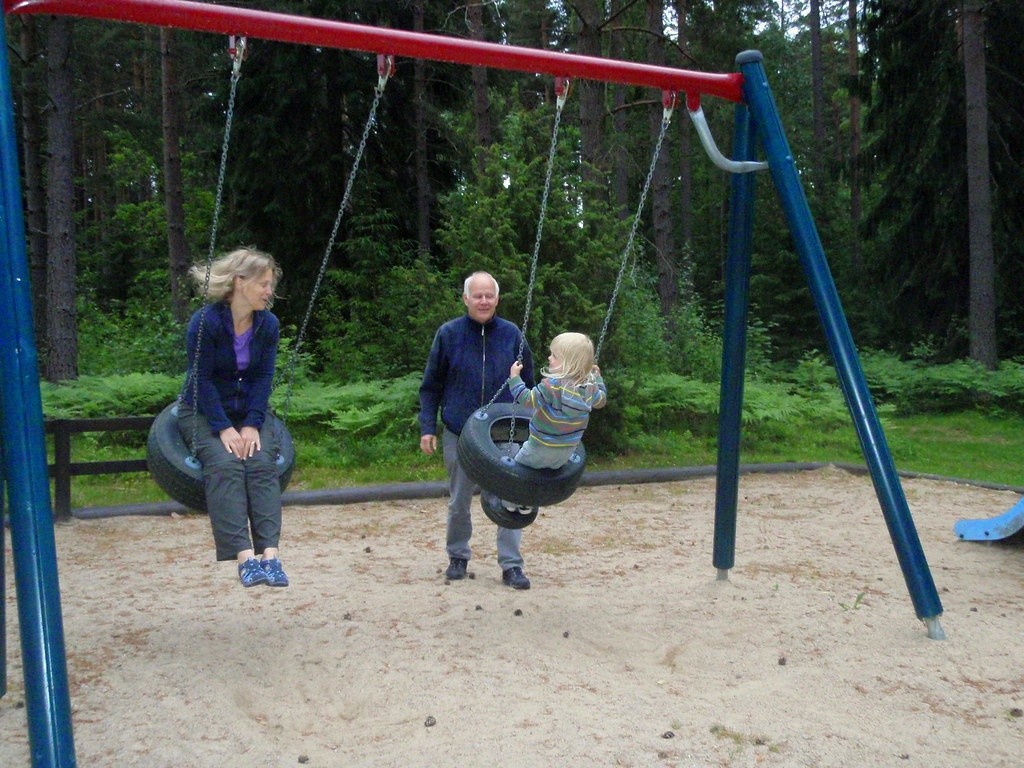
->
[260,557,289,588]
[238,558,269,588]
[503,566,531,590]
[446,557,468,580]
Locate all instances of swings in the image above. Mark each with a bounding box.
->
[144,40,397,510]
[453,81,678,509]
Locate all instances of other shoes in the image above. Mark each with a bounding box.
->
[506,507,516,512]
[519,506,532,515]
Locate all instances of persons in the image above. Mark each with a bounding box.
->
[418,269,534,591]
[176,247,291,587]
[502,331,607,519]
